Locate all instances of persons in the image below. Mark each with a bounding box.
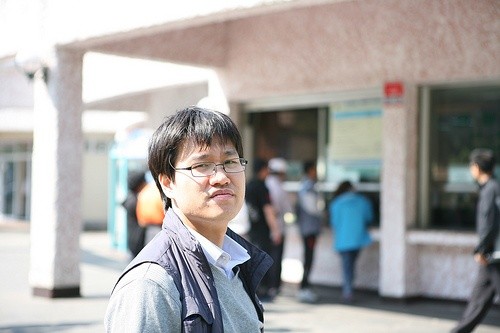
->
[264,157,288,287]
[296,161,324,303]
[121,169,147,258]
[448,148,500,332]
[102,104,273,333]
[329,182,373,299]
[245,154,278,303]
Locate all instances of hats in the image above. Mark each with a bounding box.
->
[267,158,288,172]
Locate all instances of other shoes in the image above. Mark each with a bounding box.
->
[296,288,319,302]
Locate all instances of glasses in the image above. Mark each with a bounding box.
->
[174,158,248,177]
